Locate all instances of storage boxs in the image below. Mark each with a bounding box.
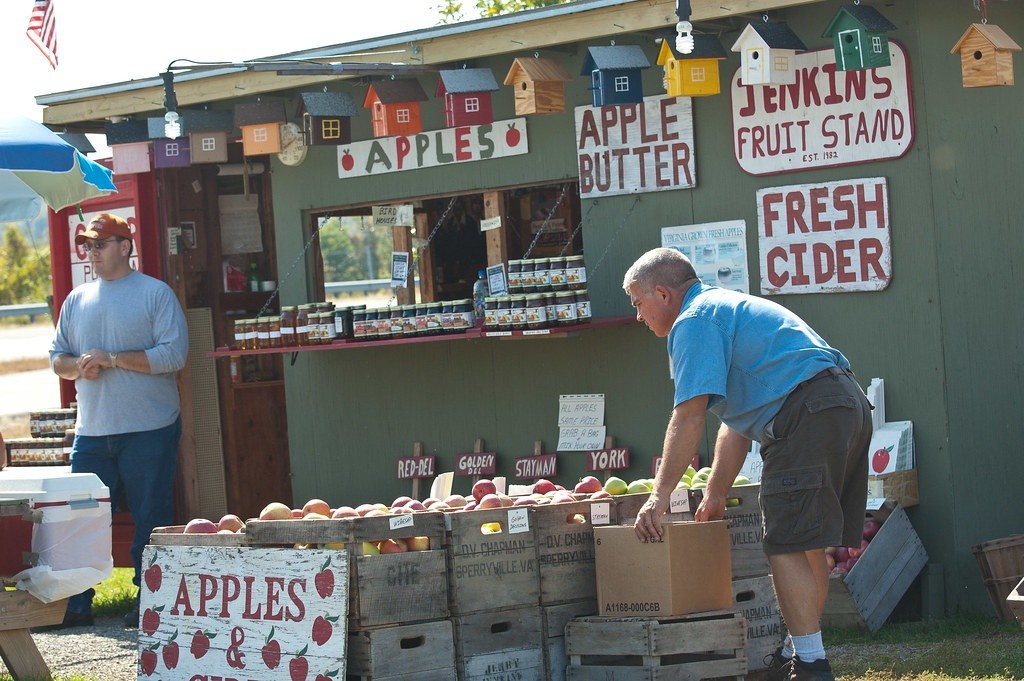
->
[609,489,693,525]
[592,518,733,617]
[0,470,113,574]
[714,575,783,671]
[246,512,451,628]
[542,600,635,681]
[531,499,617,607]
[819,498,930,635]
[346,617,460,681]
[565,612,748,681]
[422,504,542,617]
[865,468,920,507]
[453,606,547,681]
[689,483,774,581]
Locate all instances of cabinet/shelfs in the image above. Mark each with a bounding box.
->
[164,140,296,547]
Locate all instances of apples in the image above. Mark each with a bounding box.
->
[184,467,747,557]
[826,519,881,578]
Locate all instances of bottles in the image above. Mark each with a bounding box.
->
[473,272,488,319]
[233,298,472,351]
[247,264,259,291]
[2,400,78,466]
[483,255,592,332]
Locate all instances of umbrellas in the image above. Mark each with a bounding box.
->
[0,117,118,213]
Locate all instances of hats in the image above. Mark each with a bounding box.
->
[75,213,132,244]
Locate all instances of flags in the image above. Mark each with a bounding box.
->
[26,0,58,71]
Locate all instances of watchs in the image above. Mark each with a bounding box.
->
[109,352,118,368]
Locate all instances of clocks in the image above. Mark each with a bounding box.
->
[277,120,309,167]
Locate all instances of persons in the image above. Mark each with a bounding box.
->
[623,247,876,681]
[30,213,190,631]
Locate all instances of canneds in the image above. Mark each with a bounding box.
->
[482,255,591,331]
[233,299,476,351]
[3,401,78,464]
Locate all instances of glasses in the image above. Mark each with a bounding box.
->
[83,239,124,252]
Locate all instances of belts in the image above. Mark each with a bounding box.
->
[791,366,846,392]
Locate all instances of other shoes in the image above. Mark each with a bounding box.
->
[777,652,835,681]
[125,608,139,626]
[763,646,791,681]
[30,612,92,632]
